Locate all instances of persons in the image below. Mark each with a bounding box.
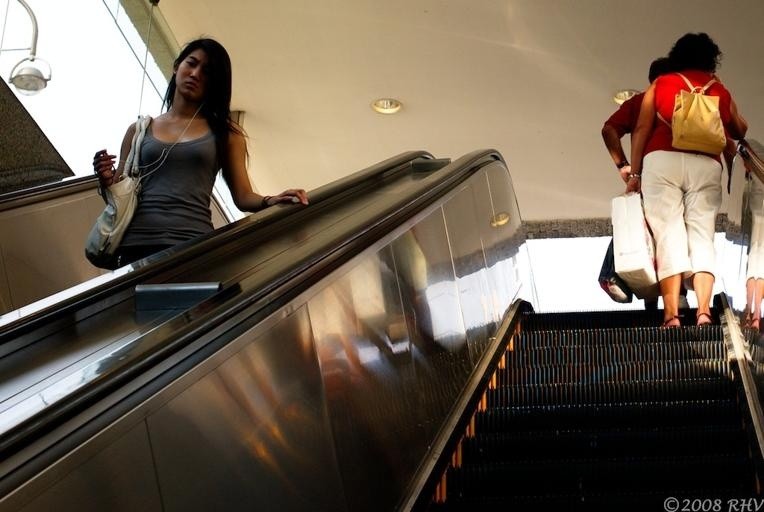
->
[602,57,689,312]
[741,163,764,331]
[626,32,748,327]
[93,39,308,266]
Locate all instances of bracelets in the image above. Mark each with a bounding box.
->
[261,196,272,206]
[628,171,641,178]
[616,160,628,168]
[97,178,114,195]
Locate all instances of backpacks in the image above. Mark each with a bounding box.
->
[670,72,728,157]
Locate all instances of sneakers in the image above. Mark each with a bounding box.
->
[659,315,680,329]
[693,313,712,327]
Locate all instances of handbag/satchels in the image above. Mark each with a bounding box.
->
[597,189,659,304]
[83,111,154,271]
